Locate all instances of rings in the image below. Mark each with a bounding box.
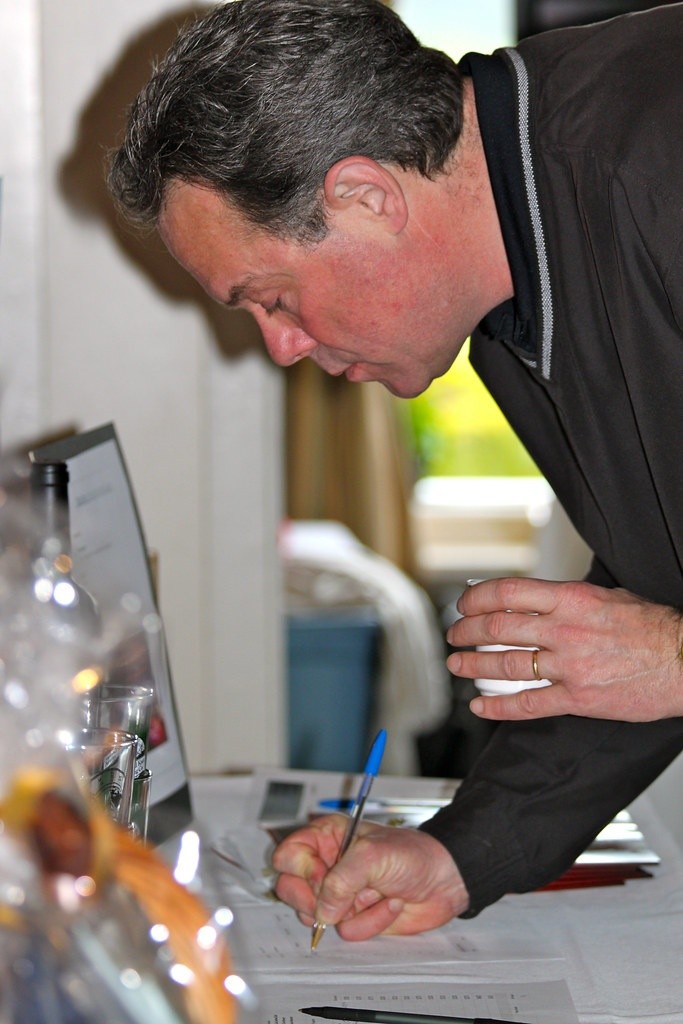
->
[532,650,542,681]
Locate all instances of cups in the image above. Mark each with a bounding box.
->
[54,677,158,851]
[463,575,561,707]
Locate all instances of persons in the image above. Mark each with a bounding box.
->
[100,0,683,944]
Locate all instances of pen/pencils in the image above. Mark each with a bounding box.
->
[308,726,389,952]
[297,1005,532,1024]
